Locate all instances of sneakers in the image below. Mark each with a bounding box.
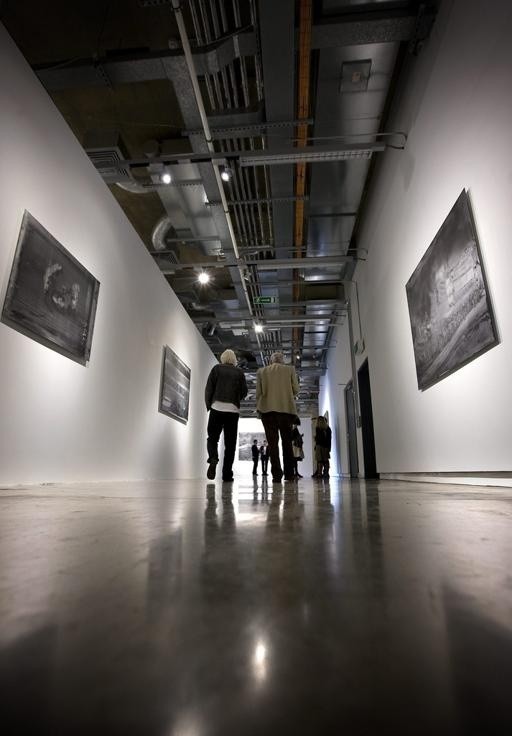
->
[314,473,329,479]
[207,462,218,479]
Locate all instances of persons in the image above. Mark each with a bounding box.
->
[203,348,249,482]
[255,353,301,484]
[251,439,262,476]
[291,414,333,481]
[259,440,269,476]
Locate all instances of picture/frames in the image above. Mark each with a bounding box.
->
[1,208,101,361]
[158,343,193,425]
